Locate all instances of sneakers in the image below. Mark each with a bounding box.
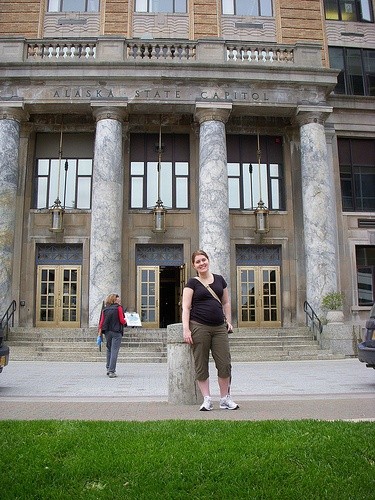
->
[200,399,215,411]
[219,398,240,411]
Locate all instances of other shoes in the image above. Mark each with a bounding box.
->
[106,370,117,378]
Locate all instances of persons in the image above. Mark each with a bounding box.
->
[98,294,126,378]
[182,251,239,411]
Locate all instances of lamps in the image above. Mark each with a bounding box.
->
[48,125,65,233]
[254,127,270,235]
[151,119,168,234]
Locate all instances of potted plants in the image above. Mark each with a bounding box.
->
[322,290,346,323]
